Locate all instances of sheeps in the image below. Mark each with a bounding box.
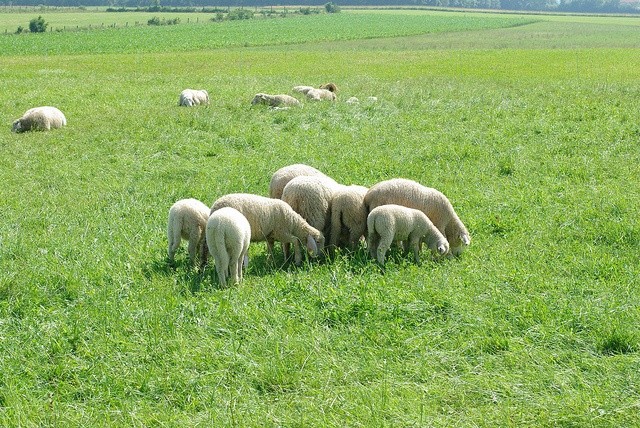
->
[306,89,337,102]
[208,193,325,267]
[367,204,450,276]
[268,160,340,199]
[364,178,472,260]
[281,175,333,261]
[178,89,209,107]
[250,93,300,106]
[11,106,67,134]
[167,198,211,274]
[328,185,369,265]
[205,207,251,290]
[291,82,340,95]
